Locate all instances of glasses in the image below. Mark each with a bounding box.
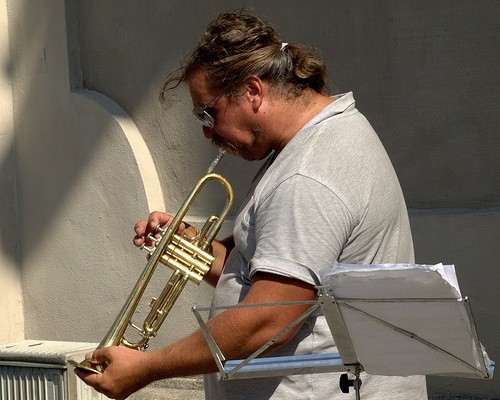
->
[193,80,237,127]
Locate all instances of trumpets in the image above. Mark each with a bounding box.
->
[66,141,235,376]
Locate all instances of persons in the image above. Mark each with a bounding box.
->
[73,6,429,400]
